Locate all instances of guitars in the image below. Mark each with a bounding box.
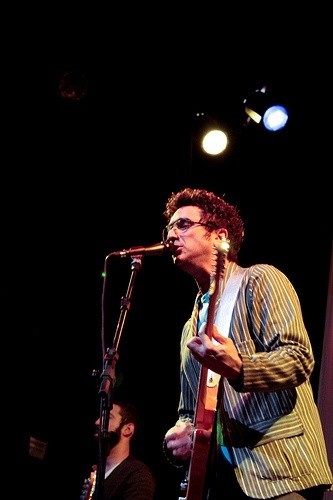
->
[80,472,96,500]
[178,239,230,499]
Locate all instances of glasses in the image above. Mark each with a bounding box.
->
[162,218,217,242]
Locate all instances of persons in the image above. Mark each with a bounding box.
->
[162,188,333,500]
[76,402,156,500]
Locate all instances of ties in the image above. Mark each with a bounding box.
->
[198,292,211,335]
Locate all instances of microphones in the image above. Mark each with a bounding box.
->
[110,241,175,258]
